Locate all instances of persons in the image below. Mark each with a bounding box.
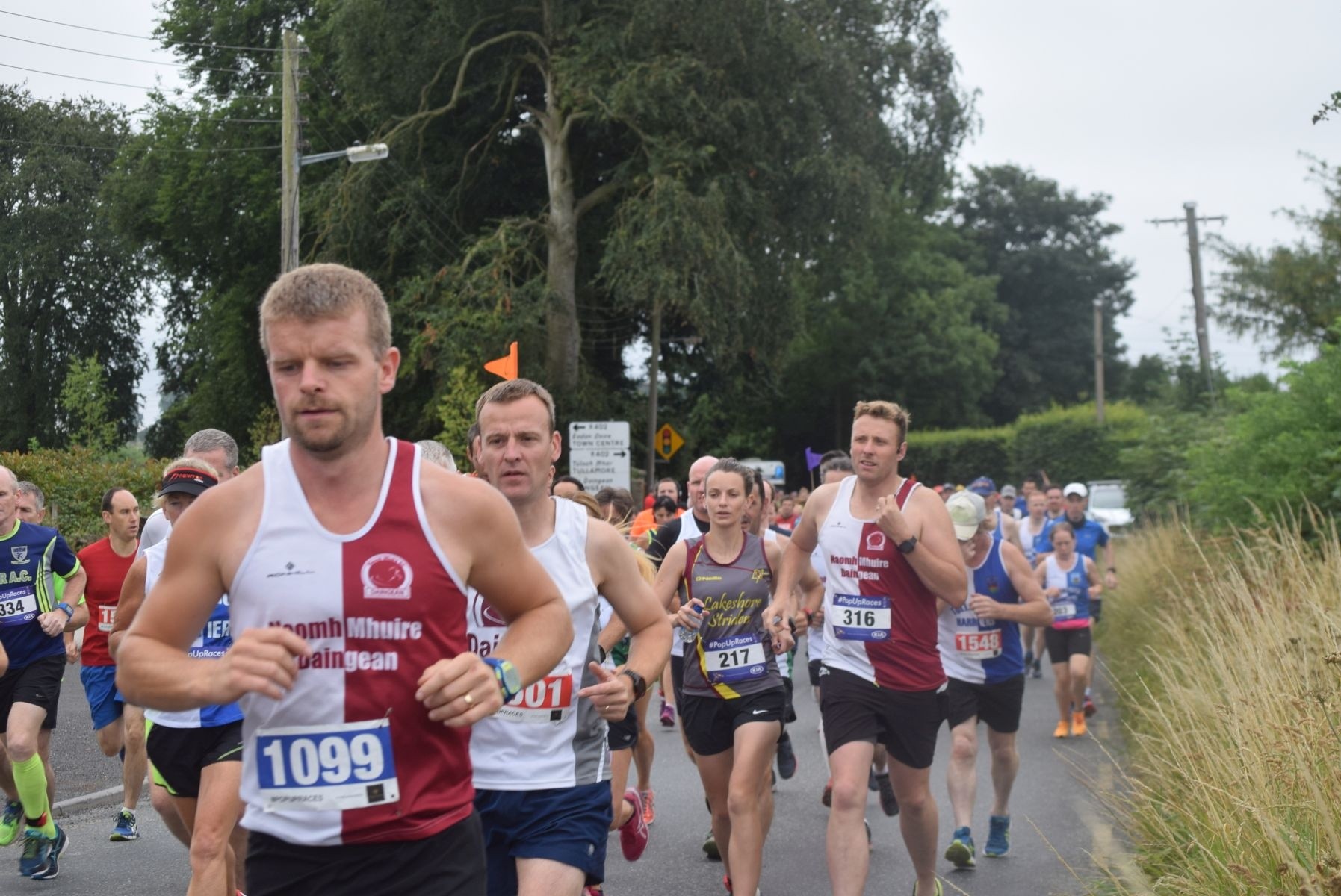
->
[418,379,676,896]
[0,428,1118,896]
[762,401,973,896]
[112,262,575,896]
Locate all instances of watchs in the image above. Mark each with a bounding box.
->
[1106,567,1116,574]
[597,645,606,664]
[481,655,523,705]
[621,669,647,701]
[56,603,73,622]
[897,536,916,554]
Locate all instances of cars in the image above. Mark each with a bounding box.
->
[1077,480,1140,535]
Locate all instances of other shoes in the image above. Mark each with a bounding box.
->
[1034,659,1042,679]
[1082,694,1095,718]
[823,785,834,807]
[875,772,898,814]
[1023,653,1035,675]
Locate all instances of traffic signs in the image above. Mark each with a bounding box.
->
[569,449,630,495]
[568,421,631,449]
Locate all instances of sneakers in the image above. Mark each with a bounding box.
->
[982,813,1011,856]
[119,745,125,762]
[703,831,721,859]
[110,811,139,840]
[661,707,675,726]
[659,700,666,715]
[19,829,57,871]
[778,733,796,780]
[1054,721,1070,737]
[30,823,69,879]
[619,790,649,861]
[0,800,25,845]
[641,789,654,824]
[1071,710,1086,734]
[945,827,978,870]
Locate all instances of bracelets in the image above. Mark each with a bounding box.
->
[800,606,813,625]
[787,616,796,634]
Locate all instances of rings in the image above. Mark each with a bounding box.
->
[463,694,476,709]
[875,507,880,511]
[604,704,610,714]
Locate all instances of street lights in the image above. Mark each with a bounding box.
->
[278,143,392,271]
[643,334,704,501]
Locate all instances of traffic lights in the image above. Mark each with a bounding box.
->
[651,424,684,460]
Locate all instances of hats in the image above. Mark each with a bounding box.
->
[971,477,995,495]
[1002,485,1017,499]
[945,491,986,540]
[1063,483,1087,498]
[157,467,219,496]
